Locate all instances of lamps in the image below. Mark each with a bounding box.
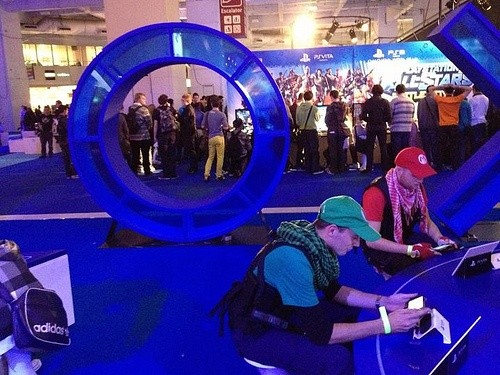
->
[323,22,339,44]
[356,22,369,31]
[349,29,358,42]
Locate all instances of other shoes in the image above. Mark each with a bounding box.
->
[215,175,226,183]
[187,166,199,176]
[158,174,180,181]
[203,175,210,182]
[312,170,326,175]
[290,167,304,172]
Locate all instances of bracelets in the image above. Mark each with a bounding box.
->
[375,295,383,308]
[407,245,414,257]
[378,306,392,334]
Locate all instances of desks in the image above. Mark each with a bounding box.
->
[288,131,382,168]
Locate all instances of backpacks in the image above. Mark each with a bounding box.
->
[156,106,178,134]
[227,130,242,159]
[127,104,148,135]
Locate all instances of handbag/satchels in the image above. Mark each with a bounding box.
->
[355,135,368,154]
[8,287,72,354]
[198,131,209,152]
[294,128,305,140]
[208,278,284,340]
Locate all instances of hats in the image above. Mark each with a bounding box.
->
[394,146,438,180]
[317,194,382,242]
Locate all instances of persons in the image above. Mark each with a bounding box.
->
[227,195,431,375]
[18,82,490,182]
[0,238,51,375]
[360,147,458,279]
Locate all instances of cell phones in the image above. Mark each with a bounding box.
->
[408,296,423,310]
[433,245,452,251]
